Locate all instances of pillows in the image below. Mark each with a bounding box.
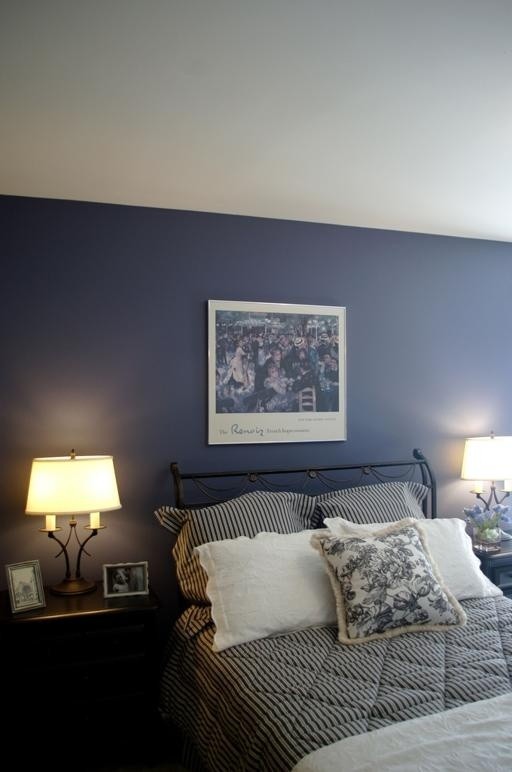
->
[193,529,339,654]
[155,490,322,643]
[321,515,504,600]
[310,480,430,529]
[309,518,467,644]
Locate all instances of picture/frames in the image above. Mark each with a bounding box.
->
[4,559,48,615]
[207,299,348,446]
[102,559,151,599]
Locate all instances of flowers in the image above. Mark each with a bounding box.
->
[462,504,512,543]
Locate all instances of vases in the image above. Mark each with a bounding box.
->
[474,526,502,553]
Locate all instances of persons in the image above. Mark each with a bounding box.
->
[125,567,140,591]
[213,311,340,414]
[113,573,129,592]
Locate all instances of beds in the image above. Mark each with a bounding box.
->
[154,447,512,771]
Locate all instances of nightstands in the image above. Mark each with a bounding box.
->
[0,580,159,629]
[470,530,512,595]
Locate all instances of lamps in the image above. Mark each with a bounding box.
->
[461,432,512,541]
[24,448,123,596]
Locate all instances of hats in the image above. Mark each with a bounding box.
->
[334,336,339,343]
[294,337,304,346]
[320,333,329,341]
